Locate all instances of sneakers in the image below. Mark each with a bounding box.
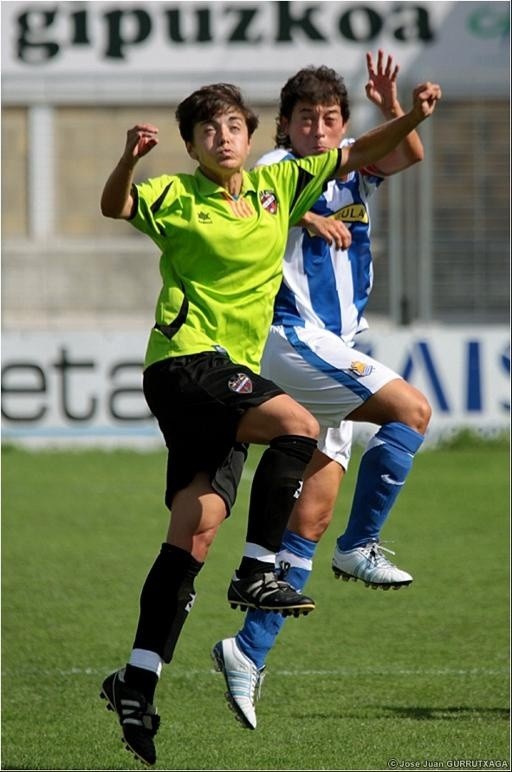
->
[99,662,161,767]
[210,637,267,730]
[227,569,315,618]
[331,536,413,590]
